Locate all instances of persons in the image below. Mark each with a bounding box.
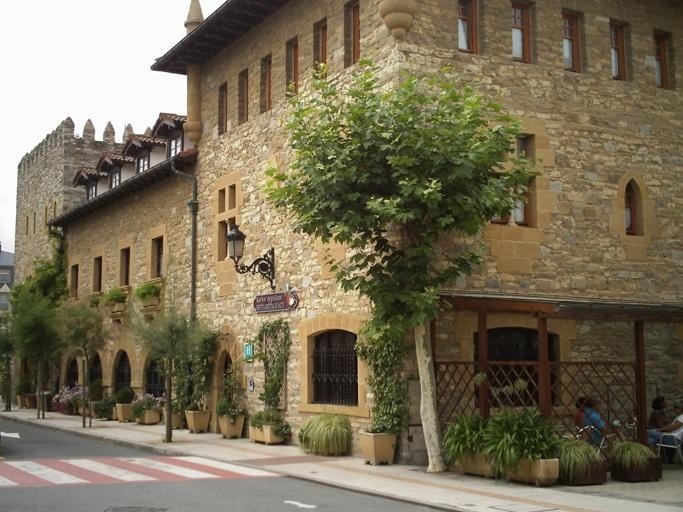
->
[581,399,624,448]
[573,396,608,432]
[647,402,682,448]
[649,395,675,464]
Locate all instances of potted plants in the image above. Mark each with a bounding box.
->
[247,320,296,444]
[441,405,657,488]
[294,408,356,460]
[350,331,409,468]
[214,357,248,442]
[14,372,160,428]
[161,332,211,433]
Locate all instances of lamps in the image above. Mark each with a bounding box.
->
[224,222,276,291]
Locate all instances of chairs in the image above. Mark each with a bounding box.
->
[655,428,683,466]
